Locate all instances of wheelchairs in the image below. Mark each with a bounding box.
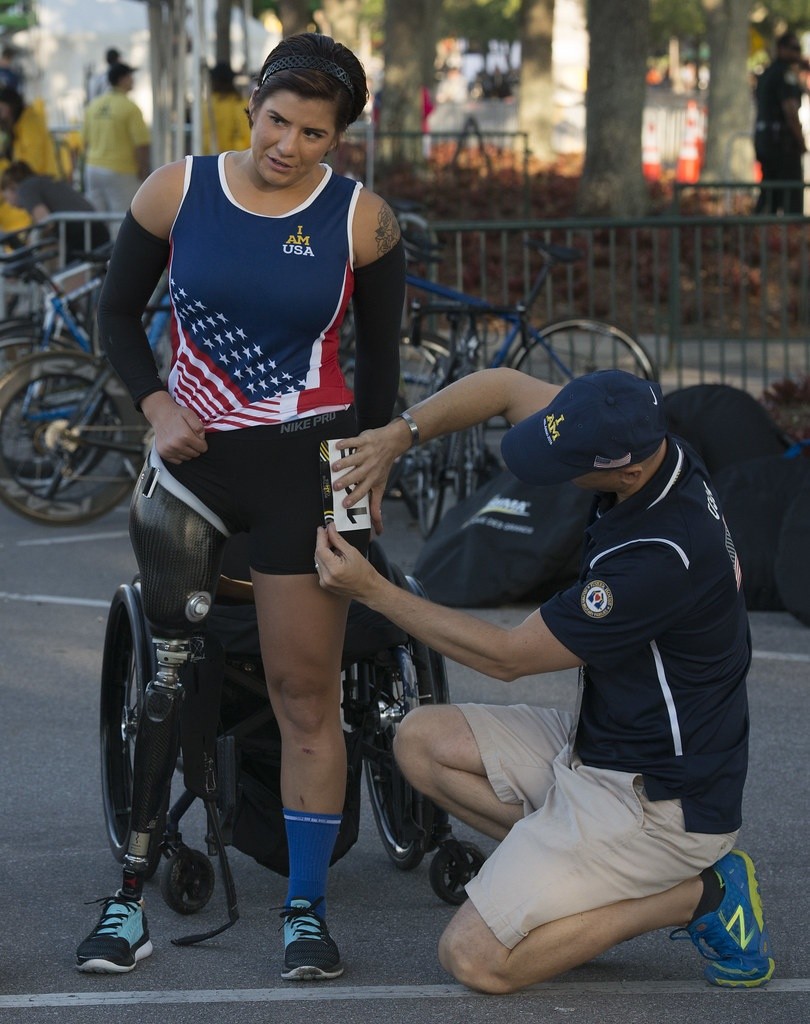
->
[97,534,489,915]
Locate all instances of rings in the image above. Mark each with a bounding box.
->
[314,564,319,569]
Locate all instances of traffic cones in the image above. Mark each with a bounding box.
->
[642,99,766,187]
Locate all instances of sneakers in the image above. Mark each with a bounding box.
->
[671,851,776,988]
[73,896,154,974]
[268,895,345,981]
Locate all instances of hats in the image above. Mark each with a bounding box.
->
[107,64,140,86]
[500,368,667,487]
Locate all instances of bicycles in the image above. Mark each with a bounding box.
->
[0,212,664,538]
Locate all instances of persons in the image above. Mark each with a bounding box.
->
[75,33,406,980]
[750,34,810,216]
[314,366,776,994]
[0,48,250,264]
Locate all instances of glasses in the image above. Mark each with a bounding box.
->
[787,44,801,52]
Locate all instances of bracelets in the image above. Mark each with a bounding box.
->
[397,412,419,447]
